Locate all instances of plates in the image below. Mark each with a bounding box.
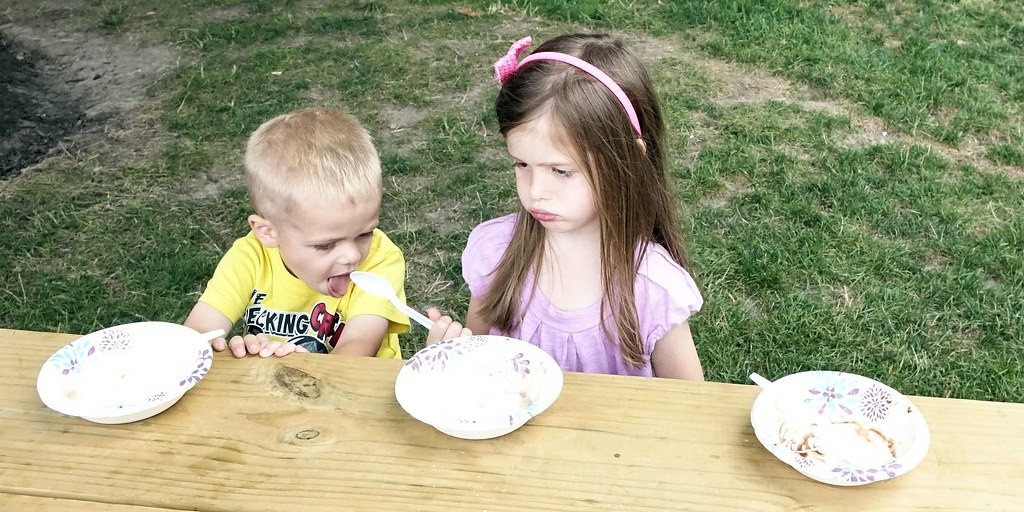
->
[395,335,563,439]
[37,321,213,425]
[751,371,930,486]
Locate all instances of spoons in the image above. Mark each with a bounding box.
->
[350,271,434,331]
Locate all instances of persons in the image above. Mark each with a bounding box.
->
[181,106,412,360]
[424,33,706,382]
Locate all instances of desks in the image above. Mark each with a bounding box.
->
[0,328,1024,512]
